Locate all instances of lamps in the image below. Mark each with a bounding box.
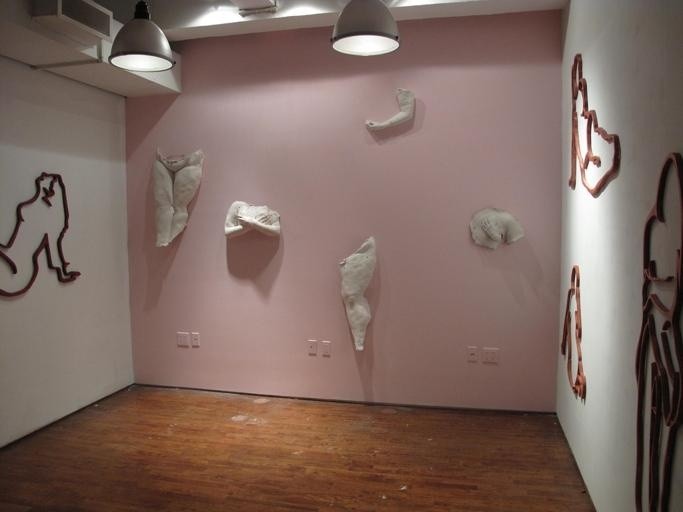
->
[332,0,400,56]
[108,0,174,72]
